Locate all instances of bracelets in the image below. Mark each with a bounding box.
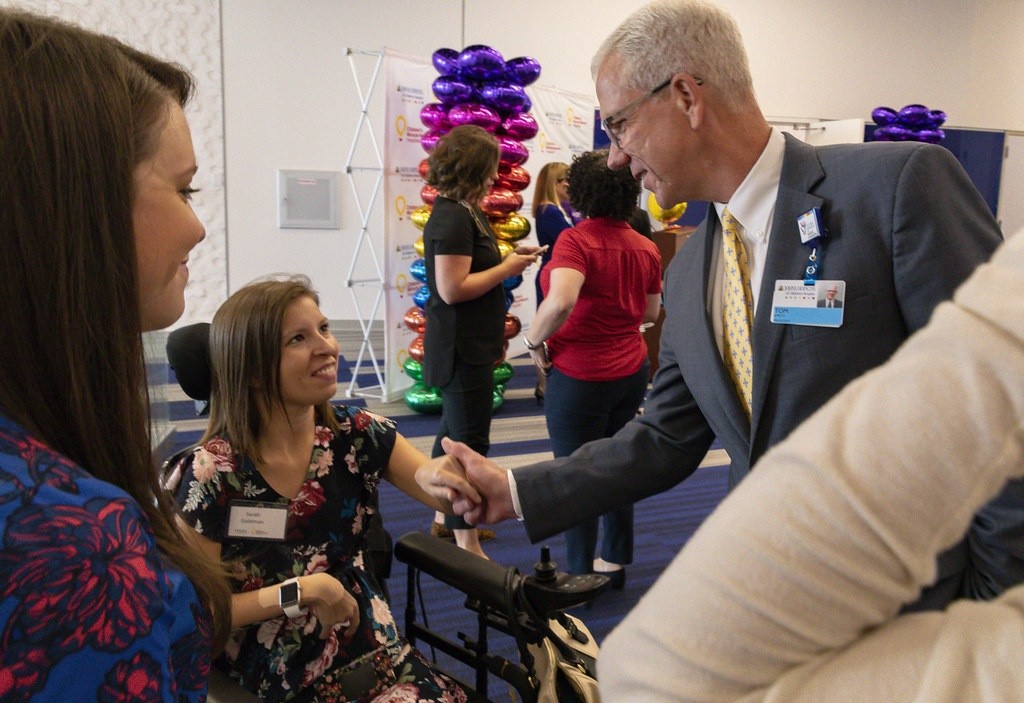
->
[523,338,539,350]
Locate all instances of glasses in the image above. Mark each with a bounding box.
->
[603,77,703,148]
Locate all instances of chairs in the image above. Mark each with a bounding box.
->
[147,320,610,703]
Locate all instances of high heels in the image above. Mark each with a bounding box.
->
[535,387,545,405]
[593,567,625,589]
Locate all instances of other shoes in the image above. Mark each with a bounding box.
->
[431,521,497,545]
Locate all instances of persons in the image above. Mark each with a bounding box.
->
[817,286,842,308]
[421,125,549,562]
[529,149,662,589]
[532,161,654,407]
[441,1,1024,617]
[593,226,1024,701]
[0,6,234,703]
[164,273,480,703]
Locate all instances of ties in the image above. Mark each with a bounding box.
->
[828,301,832,308]
[721,207,756,424]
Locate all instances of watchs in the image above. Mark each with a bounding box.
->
[279,576,308,619]
